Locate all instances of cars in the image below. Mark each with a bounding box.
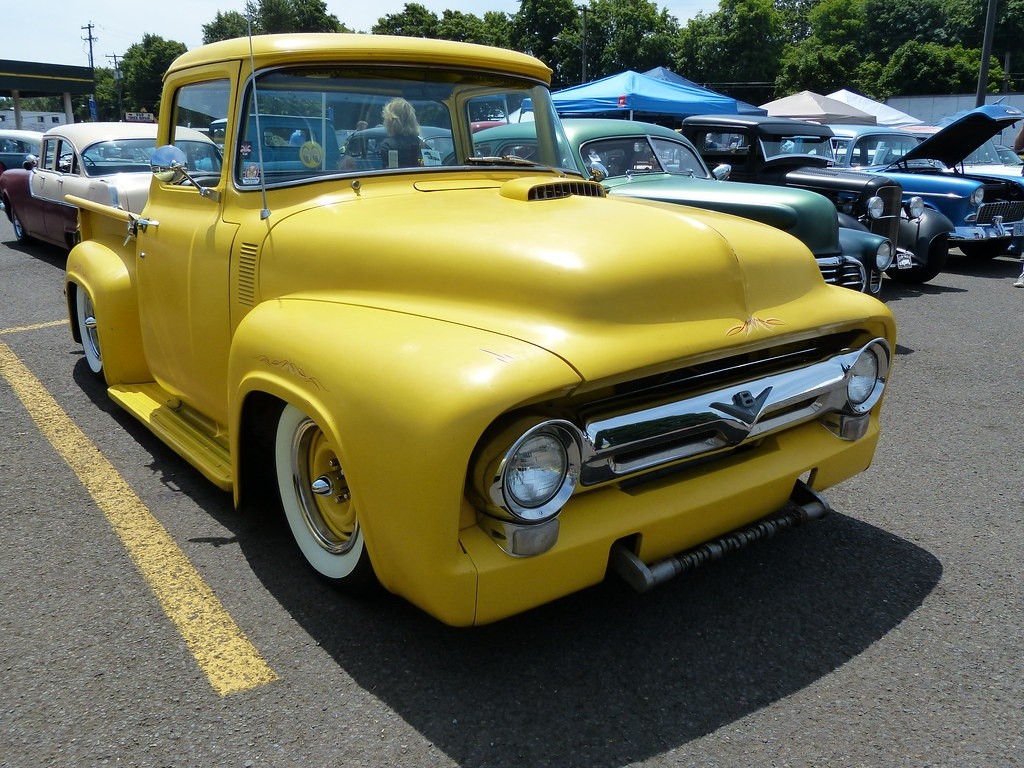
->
[0,104,1024,312]
[61,30,898,630]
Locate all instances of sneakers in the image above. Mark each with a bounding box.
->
[1014,272,1024,287]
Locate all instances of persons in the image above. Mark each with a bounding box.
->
[346,121,377,156]
[380,98,433,170]
[289,130,306,145]
[705,133,740,150]
[1014,123,1024,287]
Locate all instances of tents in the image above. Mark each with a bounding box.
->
[470,65,925,124]
